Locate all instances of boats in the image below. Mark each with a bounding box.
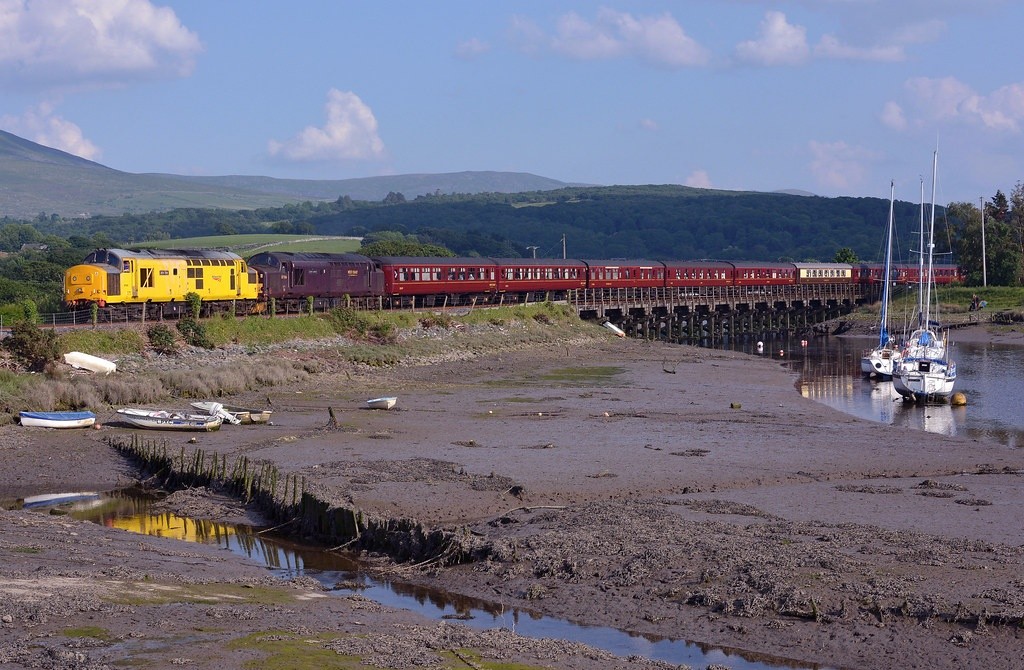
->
[366,396,398,410]
[63,350,117,377]
[19,410,96,428]
[116,406,223,432]
[189,401,275,428]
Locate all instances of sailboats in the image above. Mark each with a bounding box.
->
[861,151,957,400]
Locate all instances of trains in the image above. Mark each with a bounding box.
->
[57,248,967,320]
